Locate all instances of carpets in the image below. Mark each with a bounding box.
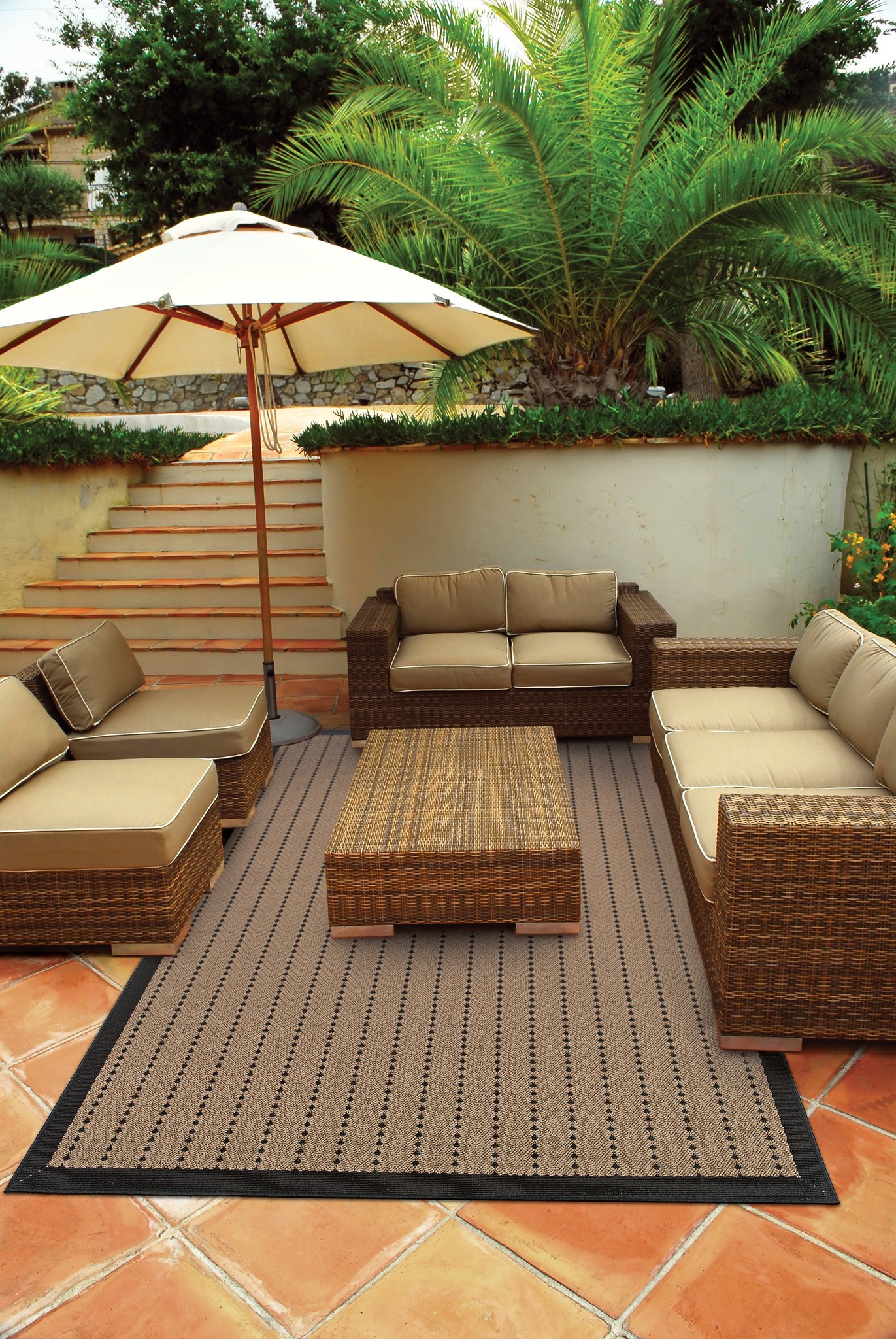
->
[3,732,843,1210]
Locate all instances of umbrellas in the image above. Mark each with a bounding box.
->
[0,201,543,719]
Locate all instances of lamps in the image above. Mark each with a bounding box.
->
[666,392,679,398]
[646,384,667,400]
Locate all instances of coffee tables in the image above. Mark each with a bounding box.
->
[321,725,582,938]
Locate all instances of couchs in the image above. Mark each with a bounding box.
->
[0,673,227,956]
[344,563,679,750]
[13,618,275,829]
[648,607,896,1054]
